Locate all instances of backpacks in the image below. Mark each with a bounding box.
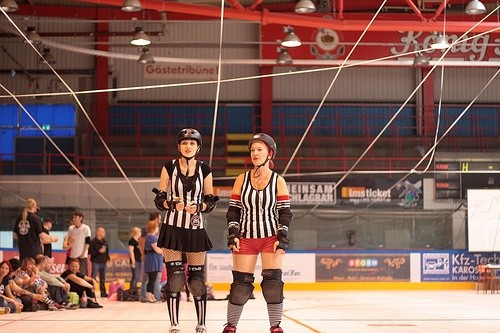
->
[109,280,124,301]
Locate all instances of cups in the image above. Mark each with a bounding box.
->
[0,306,5,315]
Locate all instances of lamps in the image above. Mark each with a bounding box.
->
[39,47,56,64]
[137,48,155,64]
[465,0,486,14]
[276,48,292,65]
[0,0,18,12]
[414,48,430,65]
[121,0,142,12]
[281,30,301,47]
[295,0,316,14]
[431,32,449,49]
[130,26,151,45]
[24,26,41,44]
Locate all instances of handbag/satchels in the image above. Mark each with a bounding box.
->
[117,287,139,301]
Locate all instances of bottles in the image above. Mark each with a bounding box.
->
[81,290,87,308]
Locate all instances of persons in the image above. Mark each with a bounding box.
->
[127,212,164,302]
[0,198,103,314]
[222,133,293,333]
[151,129,220,333]
[88,226,110,297]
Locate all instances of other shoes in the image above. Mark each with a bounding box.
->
[196,324,206,333]
[31,303,40,311]
[222,325,236,333]
[270,326,283,333]
[5,307,10,314]
[63,302,79,309]
[87,299,103,308]
[169,325,181,333]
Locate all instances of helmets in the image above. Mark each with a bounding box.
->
[176,128,202,150]
[248,133,276,159]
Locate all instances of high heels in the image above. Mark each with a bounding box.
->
[145,297,157,303]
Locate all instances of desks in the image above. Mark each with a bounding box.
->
[481,266,500,294]
[483,263,500,272]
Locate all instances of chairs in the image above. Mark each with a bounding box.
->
[477,265,499,294]
